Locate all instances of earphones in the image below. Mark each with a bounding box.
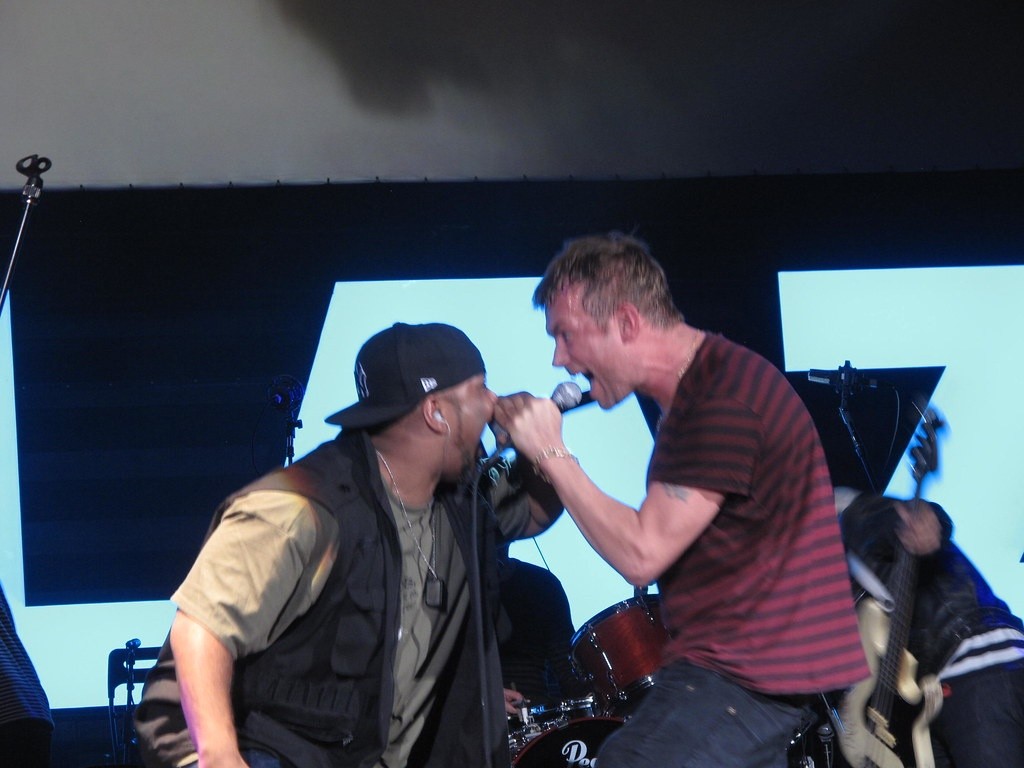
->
[432,409,447,425]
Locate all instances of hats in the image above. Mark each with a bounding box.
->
[325,322,486,429]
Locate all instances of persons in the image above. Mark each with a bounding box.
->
[833,483,1024,768]
[0,582,55,768]
[129,319,575,768]
[495,235,873,768]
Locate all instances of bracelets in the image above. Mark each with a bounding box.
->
[532,444,580,479]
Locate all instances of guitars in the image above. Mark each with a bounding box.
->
[834,406,952,768]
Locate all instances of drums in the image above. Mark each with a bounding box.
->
[568,593,678,717]
[511,716,629,768]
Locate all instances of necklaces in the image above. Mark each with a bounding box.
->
[372,448,450,615]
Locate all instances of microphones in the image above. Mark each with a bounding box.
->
[807,369,895,390]
[482,383,582,471]
